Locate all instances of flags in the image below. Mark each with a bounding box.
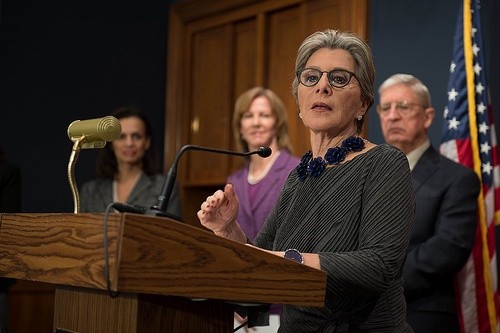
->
[436,0,500,333]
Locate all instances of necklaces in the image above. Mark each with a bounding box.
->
[296,136,371,183]
[247,151,281,185]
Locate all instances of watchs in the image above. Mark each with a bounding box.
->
[284,249,304,265]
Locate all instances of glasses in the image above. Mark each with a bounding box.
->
[296,67,362,88]
[375,100,427,115]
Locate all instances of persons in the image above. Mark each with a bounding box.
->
[197,29,416,333]
[226,87,302,333]
[376,74,481,333]
[79,104,183,221]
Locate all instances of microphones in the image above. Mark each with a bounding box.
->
[147,145,272,222]
